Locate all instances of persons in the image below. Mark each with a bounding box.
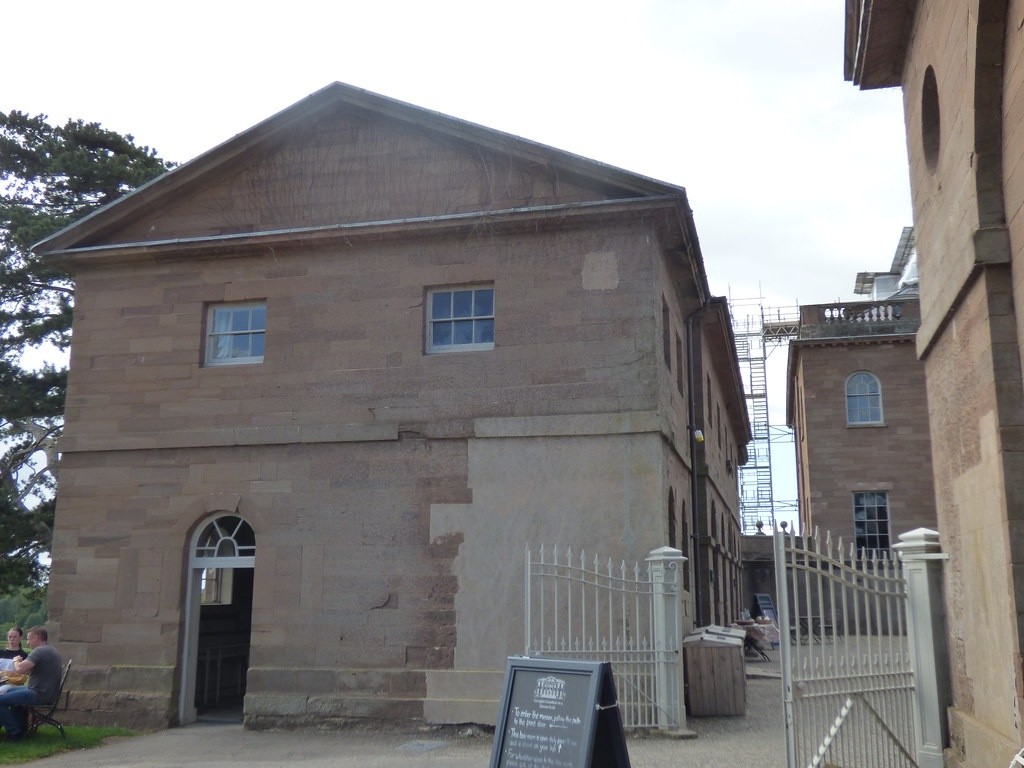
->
[0,627,29,685]
[0,626,62,741]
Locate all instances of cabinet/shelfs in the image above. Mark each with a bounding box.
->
[682,625,748,717]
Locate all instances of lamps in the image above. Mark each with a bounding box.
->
[687,423,703,444]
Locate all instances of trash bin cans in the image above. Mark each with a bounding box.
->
[683,624,747,717]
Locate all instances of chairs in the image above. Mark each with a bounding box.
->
[18,658,72,740]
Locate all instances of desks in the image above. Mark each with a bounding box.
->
[731,622,780,662]
[793,615,824,646]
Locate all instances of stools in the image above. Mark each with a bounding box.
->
[788,625,802,646]
[818,625,843,644]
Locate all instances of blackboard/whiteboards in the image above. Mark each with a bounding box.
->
[490,655,631,768]
[751,593,781,644]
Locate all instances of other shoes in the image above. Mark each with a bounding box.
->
[0,728,28,740]
